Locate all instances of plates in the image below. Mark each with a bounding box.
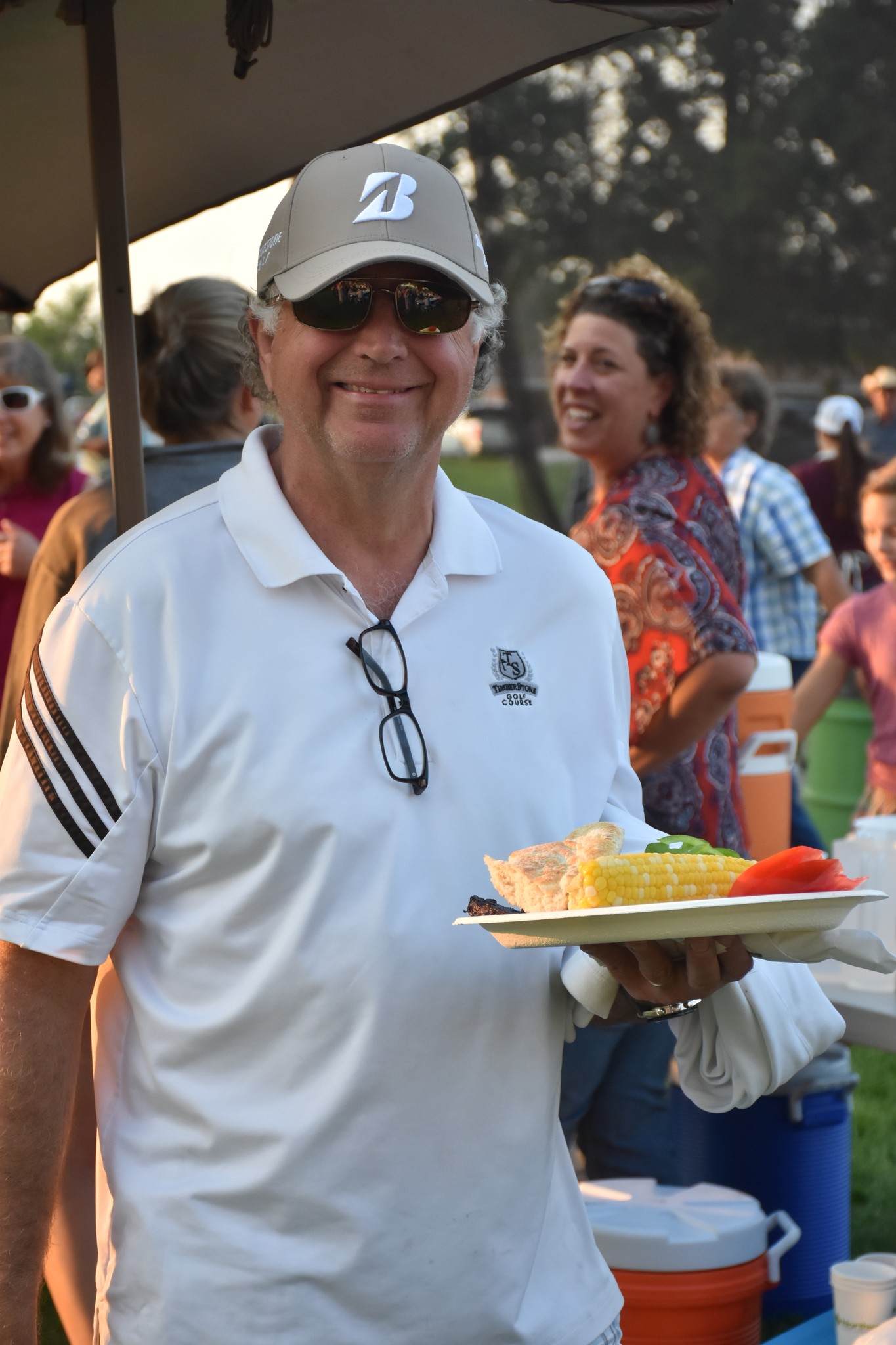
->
[451,888,889,949]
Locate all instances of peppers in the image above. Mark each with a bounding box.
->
[641,835,742,858]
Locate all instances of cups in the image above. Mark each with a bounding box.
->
[827,1251,896,1345]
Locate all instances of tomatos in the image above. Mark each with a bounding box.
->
[727,845,868,897]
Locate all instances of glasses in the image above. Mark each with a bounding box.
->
[0,385,46,411]
[347,620,429,795]
[583,276,667,313]
[264,278,480,334]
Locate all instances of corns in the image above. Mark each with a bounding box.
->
[569,853,760,909]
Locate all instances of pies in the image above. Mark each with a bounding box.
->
[484,821,624,912]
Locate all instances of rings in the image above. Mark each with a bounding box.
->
[647,970,673,987]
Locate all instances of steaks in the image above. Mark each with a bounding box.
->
[465,895,524,916]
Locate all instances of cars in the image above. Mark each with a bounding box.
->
[440,395,511,461]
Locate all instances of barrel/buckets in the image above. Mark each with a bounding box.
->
[578,1176,804,1345]
[585,1044,861,1314]
[733,651,801,866]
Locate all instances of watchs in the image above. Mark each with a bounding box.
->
[626,992,703,1023]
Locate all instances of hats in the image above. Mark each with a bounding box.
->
[82,349,103,376]
[813,394,864,436]
[861,365,896,390]
[258,143,494,307]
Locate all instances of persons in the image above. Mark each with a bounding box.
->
[0,148,649,1345]
[703,353,896,859]
[537,250,757,1189]
[0,275,268,1345]
